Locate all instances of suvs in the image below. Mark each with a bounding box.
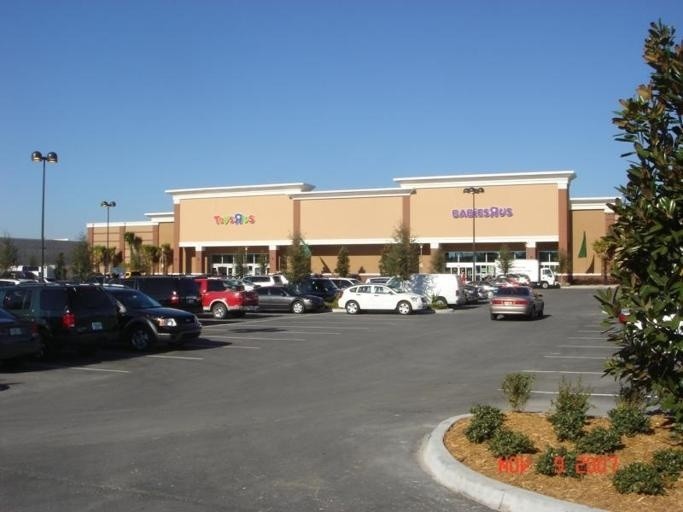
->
[0,282,120,363]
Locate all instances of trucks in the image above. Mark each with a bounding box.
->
[493,258,559,289]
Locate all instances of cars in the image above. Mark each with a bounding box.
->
[0,307,41,368]
[615,299,682,346]
[489,286,544,321]
[102,286,201,352]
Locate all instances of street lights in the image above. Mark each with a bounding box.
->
[31,151,57,283]
[462,186,484,284]
[99,201,116,283]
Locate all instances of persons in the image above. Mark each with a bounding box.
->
[106,272,124,281]
[460,270,465,286]
